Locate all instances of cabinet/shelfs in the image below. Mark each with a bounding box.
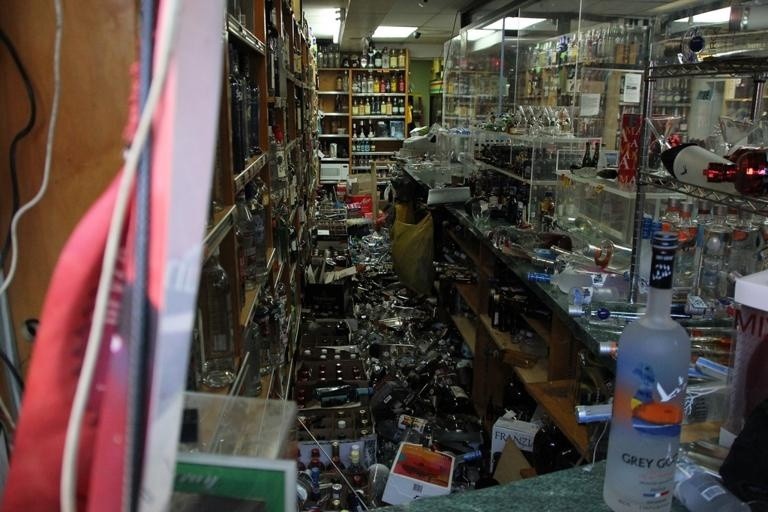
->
[1,0,768,511]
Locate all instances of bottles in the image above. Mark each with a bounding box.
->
[673,450,753,512]
[596,8,657,65]
[572,374,727,428]
[636,3,766,361]
[601,226,694,512]
[617,104,643,196]
[190,0,601,510]
[600,231,632,355]
